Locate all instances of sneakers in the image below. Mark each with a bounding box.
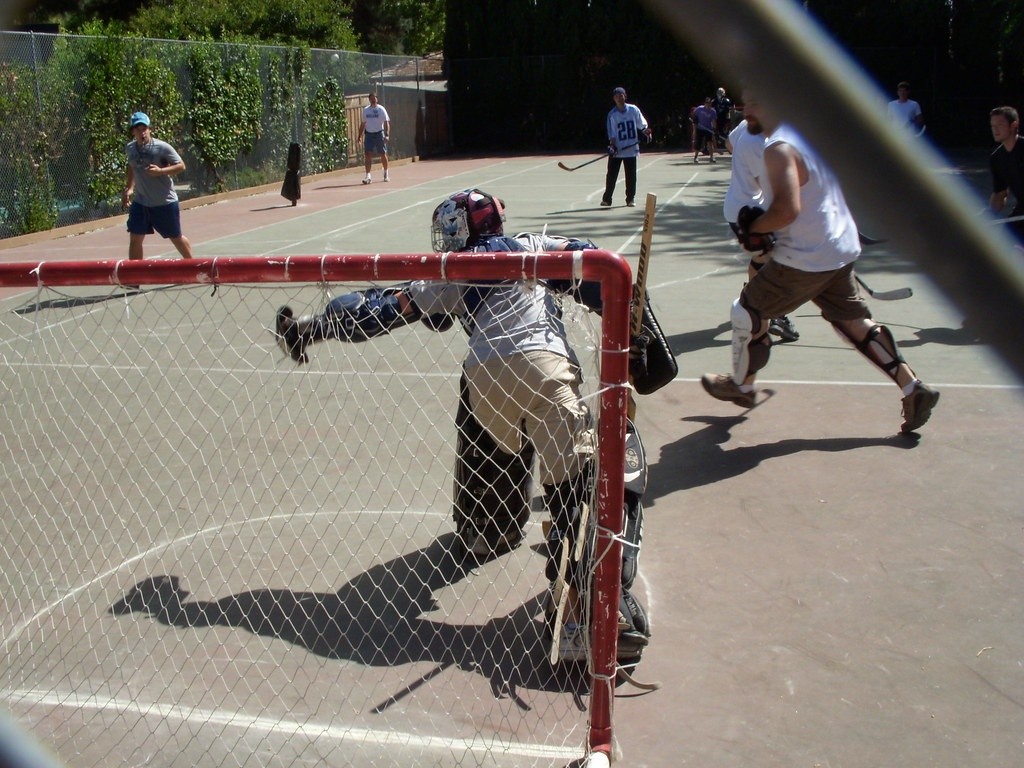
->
[769,316,800,342]
[901,378,939,434]
[700,372,756,408]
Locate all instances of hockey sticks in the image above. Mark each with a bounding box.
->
[689,117,726,141]
[857,230,891,245]
[854,273,914,301]
[541,189,659,546]
[557,139,644,172]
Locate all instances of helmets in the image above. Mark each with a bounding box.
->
[431,187,507,252]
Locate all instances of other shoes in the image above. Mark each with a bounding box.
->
[385,177,390,181]
[628,202,636,207]
[363,177,373,183]
[600,202,608,206]
[709,158,716,163]
[693,157,698,162]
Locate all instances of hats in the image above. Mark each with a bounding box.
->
[717,87,725,97]
[130,111,150,126]
[613,87,626,95]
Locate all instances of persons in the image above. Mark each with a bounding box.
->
[118,111,194,289]
[701,81,941,435]
[357,93,391,184]
[270,186,680,667]
[599,87,653,208]
[690,77,1023,341]
